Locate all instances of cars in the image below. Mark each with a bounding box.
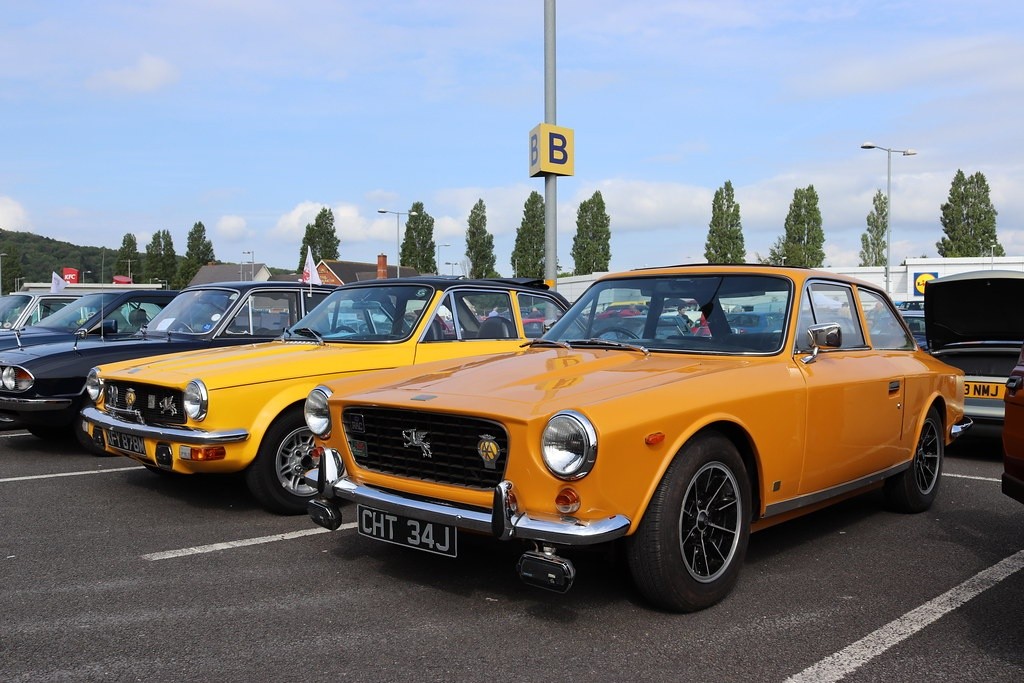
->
[305,266,974,615]
[0,282,414,456]
[79,277,596,514]
[403,275,928,348]
[0,291,290,348]
[913,269,1024,426]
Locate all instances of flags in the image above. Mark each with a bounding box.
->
[302,246,322,286]
[51,271,68,292]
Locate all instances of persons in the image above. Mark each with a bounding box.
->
[677,306,693,327]
[701,313,707,326]
[529,307,540,319]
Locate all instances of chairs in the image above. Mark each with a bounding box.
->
[726,331,784,352]
[475,316,516,341]
[420,320,441,342]
[908,305,922,310]
[10,306,35,329]
[121,309,145,333]
[908,324,921,332]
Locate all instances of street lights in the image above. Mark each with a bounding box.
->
[244,251,255,282]
[379,209,418,277]
[149,278,158,284]
[120,260,139,278]
[438,244,451,275]
[861,142,917,293]
[83,271,92,282]
[131,273,136,283]
[15,278,27,291]
[159,280,171,290]
[240,260,252,282]
[991,245,997,270]
[445,262,460,275]
